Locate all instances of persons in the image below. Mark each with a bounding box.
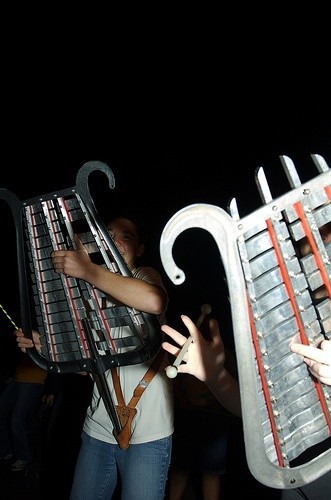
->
[161,224,331,499]
[14,215,174,500]
[2,357,55,472]
[167,374,228,499]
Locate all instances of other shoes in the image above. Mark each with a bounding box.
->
[9,459,32,471]
[0,453,13,460]
